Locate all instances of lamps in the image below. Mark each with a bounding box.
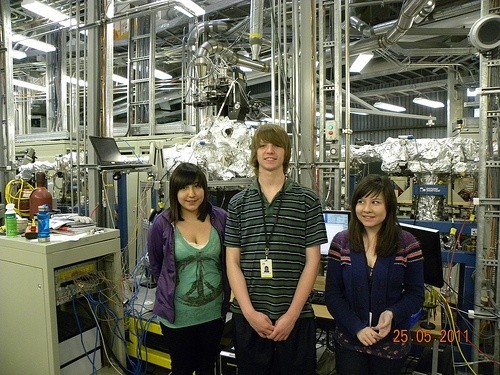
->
[10,50,27,60]
[20,0,88,36]
[63,75,89,88]
[373,101,407,113]
[413,97,445,109]
[349,54,374,72]
[11,33,56,53]
[112,73,127,84]
[13,79,47,92]
[131,63,173,80]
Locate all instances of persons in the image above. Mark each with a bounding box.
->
[147,163,227,375]
[224,123,329,375]
[324,174,425,375]
[264,266,269,272]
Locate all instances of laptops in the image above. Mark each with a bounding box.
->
[89,135,145,164]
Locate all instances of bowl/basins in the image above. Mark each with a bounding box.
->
[16,218,28,234]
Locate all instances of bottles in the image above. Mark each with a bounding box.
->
[37,204,50,243]
[5,204,17,236]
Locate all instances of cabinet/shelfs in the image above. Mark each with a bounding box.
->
[0,226,127,375]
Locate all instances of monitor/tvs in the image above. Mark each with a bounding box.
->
[318,210,352,256]
[397,221,445,288]
